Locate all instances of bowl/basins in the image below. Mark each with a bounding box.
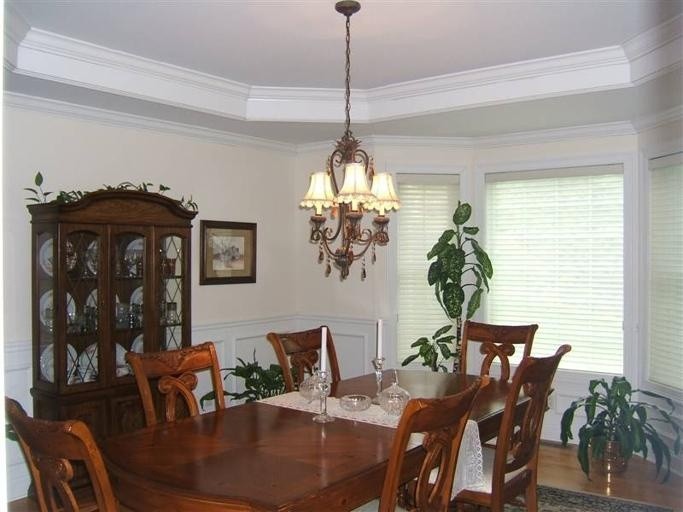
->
[339,393,371,411]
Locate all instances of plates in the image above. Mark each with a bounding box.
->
[39,290,76,329]
[86,287,121,325]
[86,238,143,276]
[129,286,144,320]
[39,238,78,277]
[40,333,143,383]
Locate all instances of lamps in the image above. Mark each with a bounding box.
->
[297,0,404,282]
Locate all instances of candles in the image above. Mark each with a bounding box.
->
[376,318,382,359]
[319,325,327,378]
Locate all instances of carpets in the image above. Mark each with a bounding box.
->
[449,483,674,511]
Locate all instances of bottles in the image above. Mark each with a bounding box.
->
[378,382,410,416]
[165,301,180,326]
[298,374,331,401]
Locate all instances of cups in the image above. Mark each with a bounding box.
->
[166,258,177,275]
[115,303,129,328]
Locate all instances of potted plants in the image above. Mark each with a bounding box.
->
[558,373,683,486]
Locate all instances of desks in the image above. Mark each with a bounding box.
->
[94,367,555,512]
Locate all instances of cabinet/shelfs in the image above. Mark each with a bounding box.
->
[25,189,199,509]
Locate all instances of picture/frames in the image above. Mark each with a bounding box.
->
[198,219,258,287]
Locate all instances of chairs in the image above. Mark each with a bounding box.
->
[123,339,227,427]
[405,342,572,511]
[459,317,539,382]
[265,324,341,393]
[4,394,118,511]
[349,373,492,511]
